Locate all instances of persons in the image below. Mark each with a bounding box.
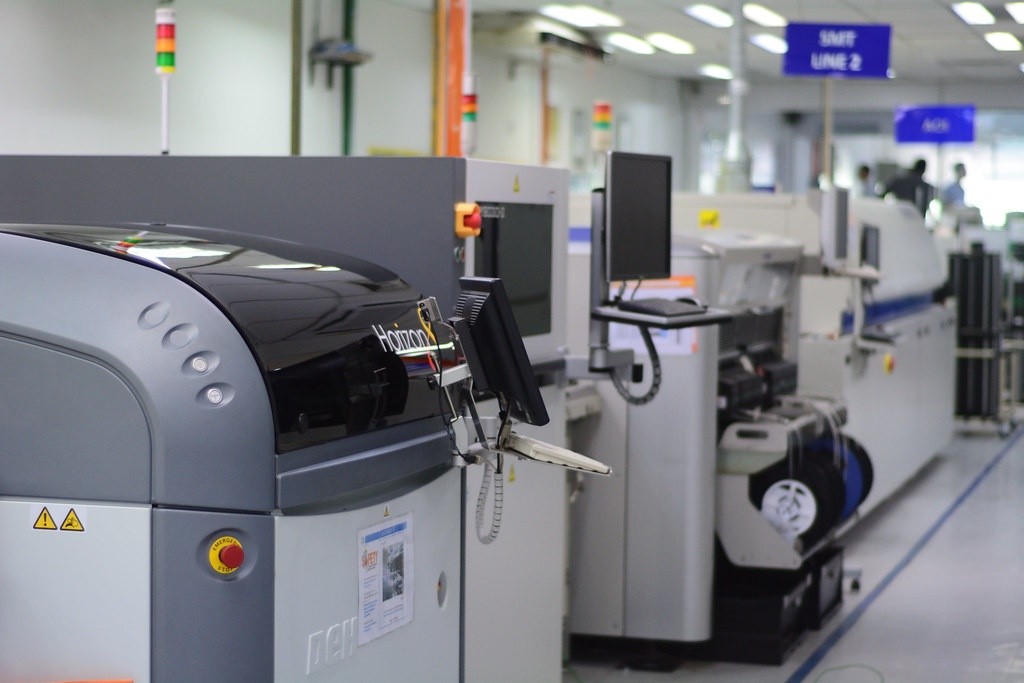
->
[858,166,876,197]
[883,160,933,214]
[942,164,966,209]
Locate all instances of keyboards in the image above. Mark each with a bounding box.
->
[506,433,609,474]
[618,298,706,318]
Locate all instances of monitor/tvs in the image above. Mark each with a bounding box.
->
[835,186,849,260]
[859,225,880,286]
[604,151,672,282]
[456,275,550,425]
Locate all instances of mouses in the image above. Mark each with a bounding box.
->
[676,297,701,306]
